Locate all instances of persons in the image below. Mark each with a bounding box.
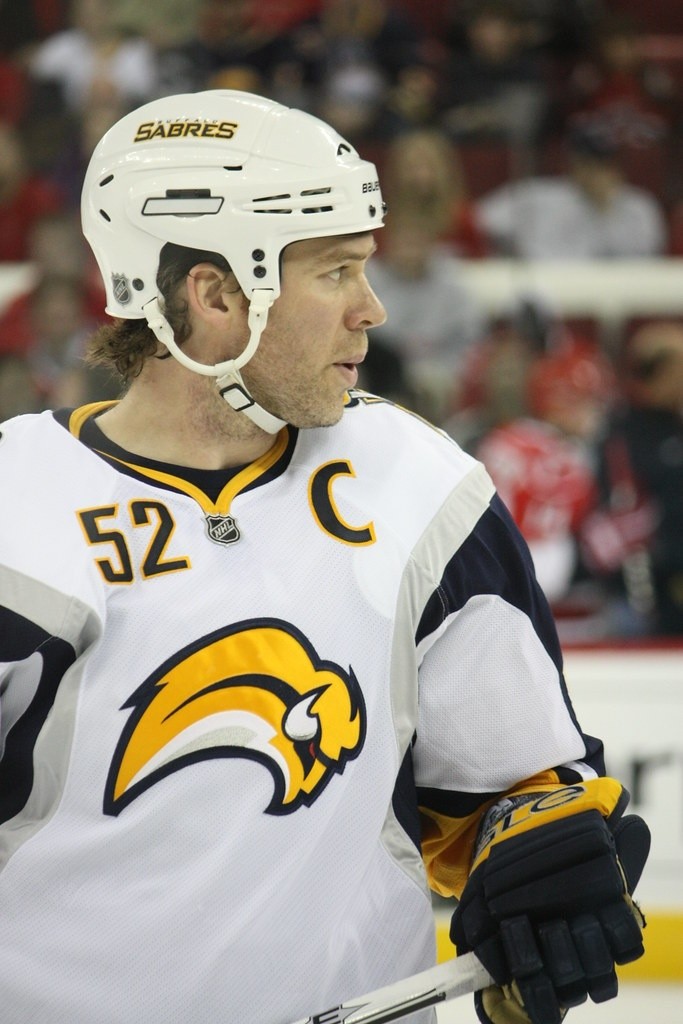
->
[0,0,683,645]
[0,90,652,1024]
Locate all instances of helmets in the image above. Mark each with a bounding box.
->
[78,87,383,320]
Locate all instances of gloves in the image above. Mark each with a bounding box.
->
[450,780,651,1024]
